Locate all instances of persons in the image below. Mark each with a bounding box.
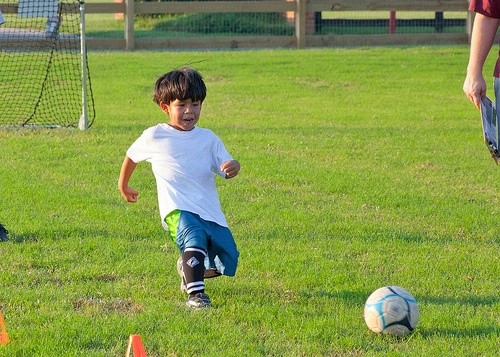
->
[462,0,500,162]
[118,65,241,308]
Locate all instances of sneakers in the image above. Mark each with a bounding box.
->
[176,257,188,294]
[185,293,213,308]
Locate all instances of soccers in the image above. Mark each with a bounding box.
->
[363,285,419,342]
